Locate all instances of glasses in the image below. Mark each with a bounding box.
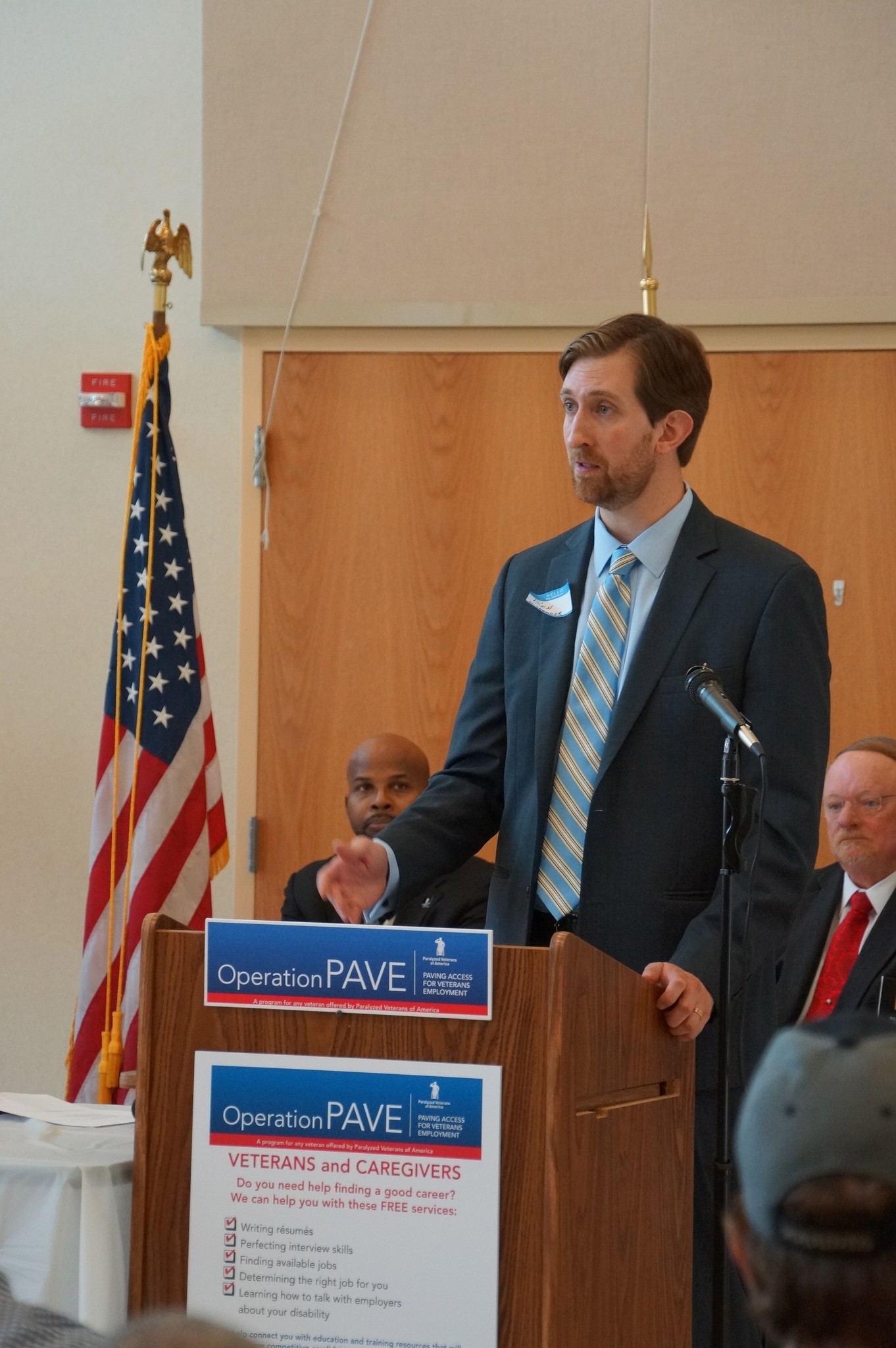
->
[821,791,896,820]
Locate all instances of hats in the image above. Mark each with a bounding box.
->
[735,1023,896,1252]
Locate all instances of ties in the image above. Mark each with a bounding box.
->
[803,891,873,1023]
[535,546,641,920]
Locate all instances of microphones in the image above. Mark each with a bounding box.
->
[685,669,765,759]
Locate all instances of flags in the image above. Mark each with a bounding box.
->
[66,322,225,1104]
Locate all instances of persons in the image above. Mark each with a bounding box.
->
[777,731,896,1044]
[280,734,497,930]
[720,1013,896,1348]
[314,313,832,1348]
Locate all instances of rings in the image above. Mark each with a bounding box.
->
[694,1008,702,1020]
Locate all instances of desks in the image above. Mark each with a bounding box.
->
[0,1104,136,1339]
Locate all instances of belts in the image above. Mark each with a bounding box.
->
[531,892,579,917]
